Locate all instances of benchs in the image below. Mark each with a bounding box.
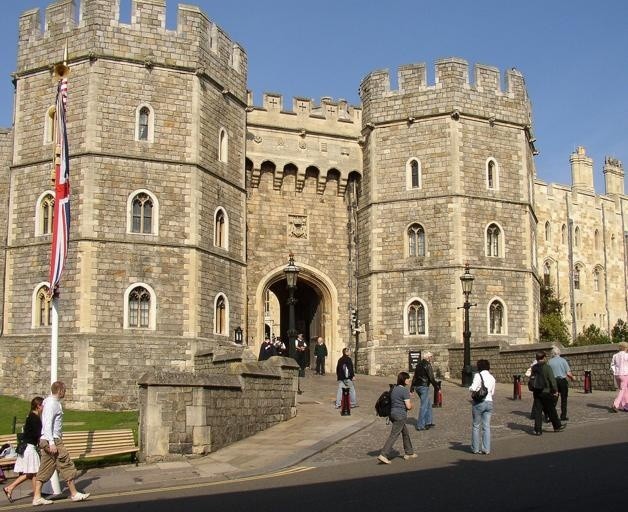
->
[0,428,139,482]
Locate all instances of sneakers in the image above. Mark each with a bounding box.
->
[70,491,91,502]
[31,495,54,507]
[402,452,419,460]
[416,423,436,431]
[376,454,393,465]
[545,417,552,423]
[607,407,618,413]
[560,416,569,421]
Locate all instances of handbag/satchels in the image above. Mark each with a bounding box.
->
[471,372,489,403]
[15,431,30,455]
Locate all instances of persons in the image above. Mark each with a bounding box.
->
[410,350,442,431]
[525,360,548,419]
[2,397,44,503]
[335,347,359,409]
[532,351,567,436]
[32,381,91,506]
[545,346,576,423]
[259,332,328,378]
[377,372,418,464]
[610,342,628,413]
[468,359,495,455]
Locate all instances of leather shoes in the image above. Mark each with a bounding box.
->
[536,429,543,436]
[553,423,568,432]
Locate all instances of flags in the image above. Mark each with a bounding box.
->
[49,78,70,295]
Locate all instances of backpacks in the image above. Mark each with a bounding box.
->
[527,362,546,393]
[375,383,397,418]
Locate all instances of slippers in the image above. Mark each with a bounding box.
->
[3,486,15,504]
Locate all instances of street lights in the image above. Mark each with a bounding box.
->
[455,259,479,390]
[282,249,302,395]
[351,307,362,374]
